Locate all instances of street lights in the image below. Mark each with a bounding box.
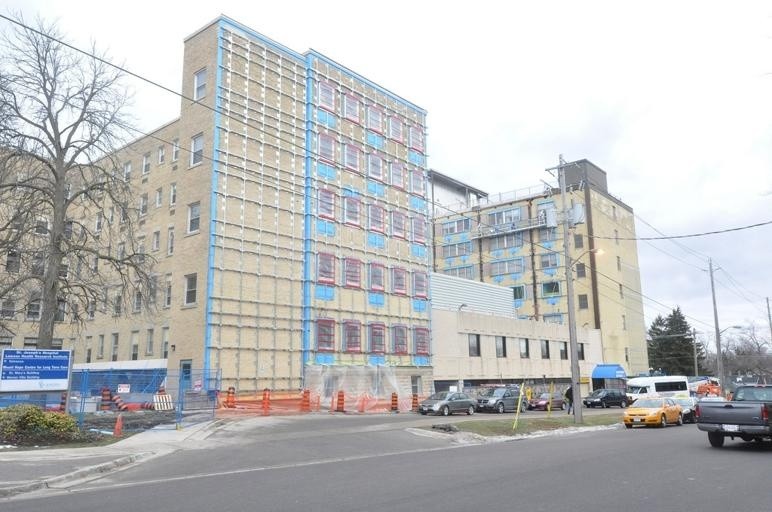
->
[563,246,606,424]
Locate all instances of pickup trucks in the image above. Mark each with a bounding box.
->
[695,382,772,446]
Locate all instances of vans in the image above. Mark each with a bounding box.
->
[625,374,690,404]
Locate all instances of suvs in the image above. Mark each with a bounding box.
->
[476,383,528,413]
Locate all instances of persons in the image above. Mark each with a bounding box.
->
[564,387,575,416]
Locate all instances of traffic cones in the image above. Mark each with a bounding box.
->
[330,396,336,414]
[113,413,124,438]
[317,396,321,411]
[216,390,227,409]
[358,397,367,411]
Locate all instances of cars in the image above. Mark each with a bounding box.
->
[417,390,479,416]
[623,396,683,428]
[582,388,629,408]
[677,396,700,423]
[528,392,567,410]
